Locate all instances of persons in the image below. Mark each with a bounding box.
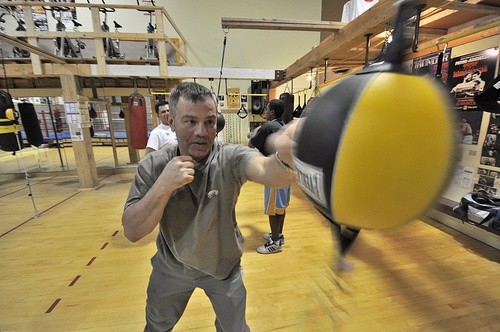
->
[143,101,178,156]
[246,99,293,254]
[461,116,474,144]
[122,82,303,331]
[463,73,472,83]
[472,70,485,91]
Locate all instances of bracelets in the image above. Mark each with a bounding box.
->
[275,151,293,171]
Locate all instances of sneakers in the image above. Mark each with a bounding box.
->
[263,233,284,244]
[256,238,281,253]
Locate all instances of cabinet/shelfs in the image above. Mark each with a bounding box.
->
[461,192,500,228]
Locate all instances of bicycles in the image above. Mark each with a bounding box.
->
[0,0,44,59]
[87,0,122,60]
[40,2,86,59]
[137,0,160,60]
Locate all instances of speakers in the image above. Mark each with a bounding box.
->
[274,70,285,81]
[251,79,263,114]
